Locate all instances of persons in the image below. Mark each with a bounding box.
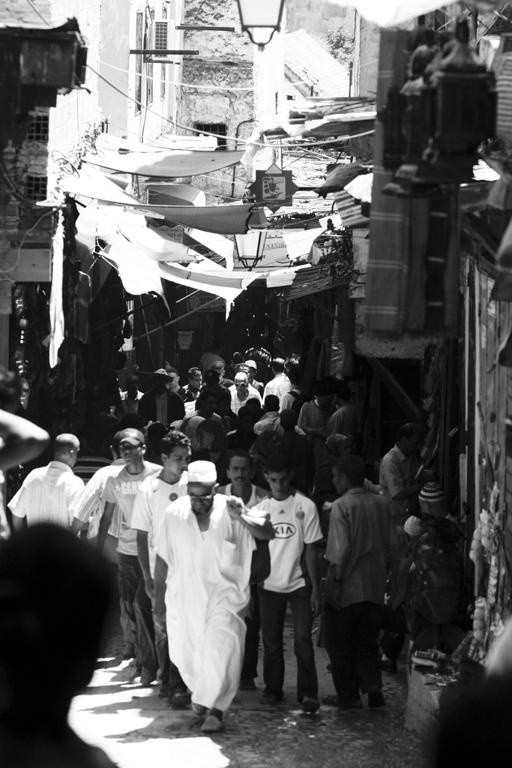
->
[152,458,275,734]
[1,330,464,711]
[1,518,123,768]
[423,677,511,765]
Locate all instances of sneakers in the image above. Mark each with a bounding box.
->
[239,679,258,690]
[260,690,283,704]
[301,701,319,712]
[121,651,206,713]
[323,695,362,709]
[368,691,385,706]
[380,659,397,672]
[201,715,224,732]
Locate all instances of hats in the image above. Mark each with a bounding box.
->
[151,368,173,381]
[417,481,447,507]
[187,460,217,484]
[117,428,146,447]
